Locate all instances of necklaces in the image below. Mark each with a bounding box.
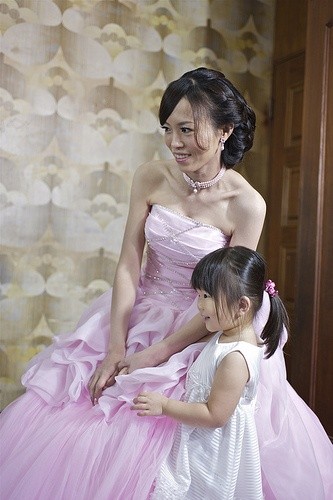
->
[182,162,226,193]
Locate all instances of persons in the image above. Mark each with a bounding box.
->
[1,65,332,500]
[131,246,288,500]
